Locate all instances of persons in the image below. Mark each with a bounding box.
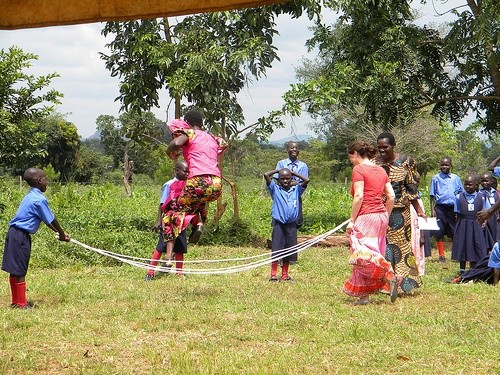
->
[341,140,400,305]
[409,171,426,275]
[263,167,310,281]
[145,161,190,279]
[451,155,500,285]
[1,168,71,308]
[429,156,465,261]
[370,134,427,292]
[161,110,230,269]
[273,140,309,229]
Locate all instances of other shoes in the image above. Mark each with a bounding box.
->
[446,278,460,284]
[160,262,171,275]
[188,226,204,244]
[270,274,291,281]
[353,301,369,306]
[11,302,39,309]
[439,259,447,264]
[147,274,156,279]
[389,276,404,303]
